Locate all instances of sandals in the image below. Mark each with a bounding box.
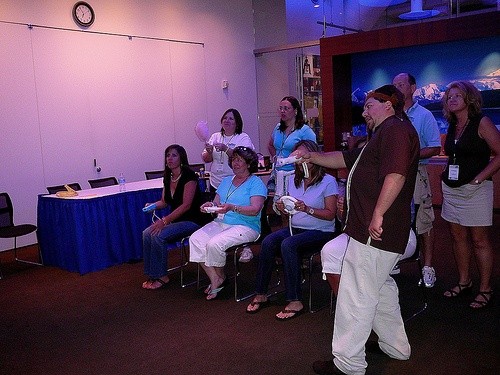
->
[469,289,494,309]
[444,280,473,297]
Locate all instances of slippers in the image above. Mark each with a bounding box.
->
[142,278,156,289]
[246,299,270,314]
[275,309,304,320]
[147,278,171,291]
[203,275,229,295]
[205,286,224,301]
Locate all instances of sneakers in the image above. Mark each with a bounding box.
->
[418,266,436,288]
[239,247,253,263]
[390,263,400,275]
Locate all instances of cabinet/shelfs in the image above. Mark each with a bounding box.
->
[296,54,325,151]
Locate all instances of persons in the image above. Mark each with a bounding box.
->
[189,146,267,301]
[201,109,256,263]
[246,140,339,321]
[142,145,207,289]
[442,80,500,310]
[266,96,317,228]
[288,85,420,375]
[389,73,442,288]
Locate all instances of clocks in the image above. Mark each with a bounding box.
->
[72,1,95,26]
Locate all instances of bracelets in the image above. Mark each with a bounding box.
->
[232,205,237,212]
[472,178,481,184]
[210,202,214,207]
[162,217,167,225]
[307,206,316,217]
[224,146,230,153]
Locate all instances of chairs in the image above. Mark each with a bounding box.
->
[88,177,118,189]
[167,194,431,329]
[0,193,44,278]
[47,183,82,194]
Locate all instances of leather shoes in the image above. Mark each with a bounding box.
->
[313,360,347,375]
[365,341,383,353]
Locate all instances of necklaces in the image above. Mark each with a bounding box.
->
[170,172,181,182]
[304,175,317,184]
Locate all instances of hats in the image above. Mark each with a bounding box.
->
[366,85,405,111]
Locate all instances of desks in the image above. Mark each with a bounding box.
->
[36,175,206,276]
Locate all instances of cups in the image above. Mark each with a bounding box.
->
[263,155,270,171]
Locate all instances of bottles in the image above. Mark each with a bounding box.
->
[118,172,127,192]
[200,168,205,178]
